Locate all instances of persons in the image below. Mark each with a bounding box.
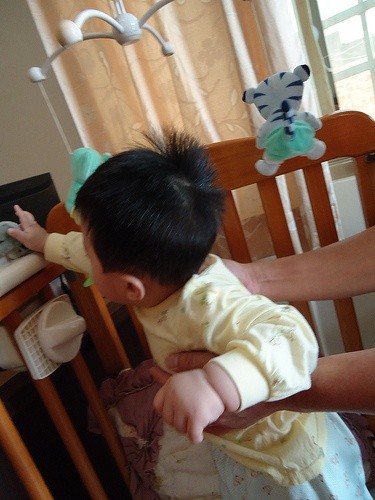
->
[7,126,375,500]
[150,222,375,432]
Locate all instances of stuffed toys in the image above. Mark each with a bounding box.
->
[65,147,111,215]
[242,65,326,176]
